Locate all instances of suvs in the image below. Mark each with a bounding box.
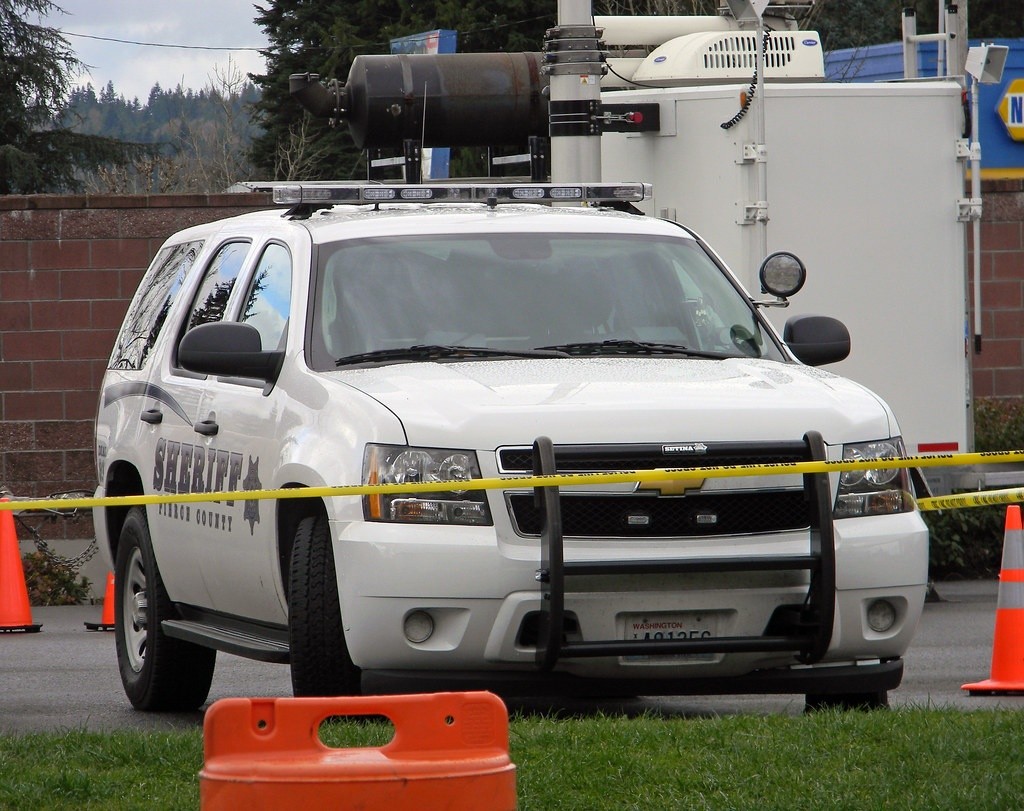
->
[92,177,931,714]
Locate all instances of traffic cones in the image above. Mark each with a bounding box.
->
[959,504,1024,698]
[82,571,115,631]
[0,497,43,634]
